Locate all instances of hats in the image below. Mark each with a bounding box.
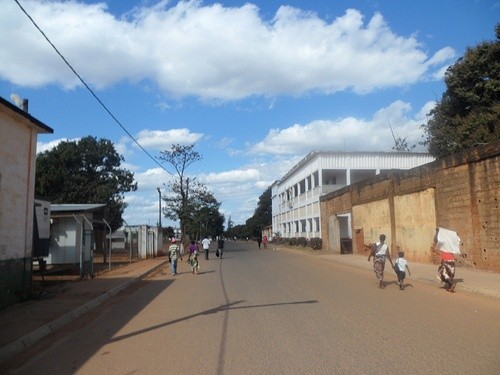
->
[172,239,176,242]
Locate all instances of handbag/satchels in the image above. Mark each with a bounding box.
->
[169,257,171,263]
[216,249,219,257]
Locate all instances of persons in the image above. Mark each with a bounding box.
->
[263,235,268,248]
[257,235,261,248]
[187,241,200,275]
[393,252,411,290]
[434,227,460,291]
[218,235,224,258]
[201,236,211,260]
[367,233,393,289]
[168,239,183,276]
